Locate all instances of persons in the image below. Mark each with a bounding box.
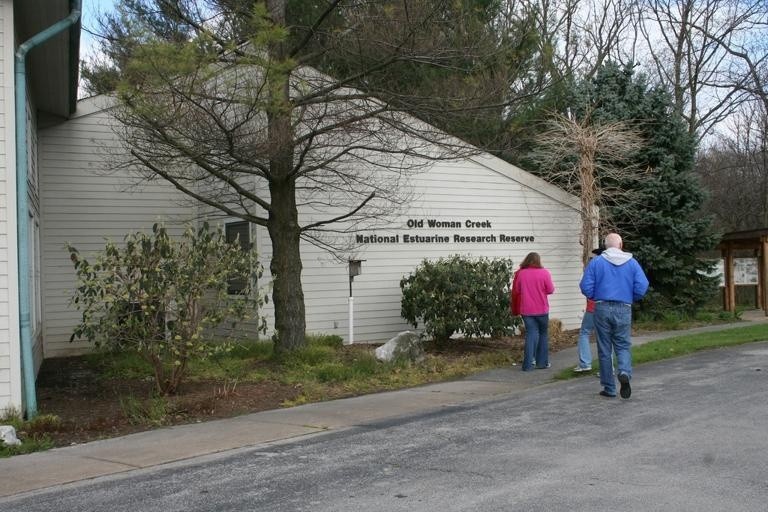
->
[572,248,602,377]
[579,233,649,399]
[509,252,554,372]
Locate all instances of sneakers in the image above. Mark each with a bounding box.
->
[617,371,631,398]
[600,391,616,397]
[574,365,592,371]
